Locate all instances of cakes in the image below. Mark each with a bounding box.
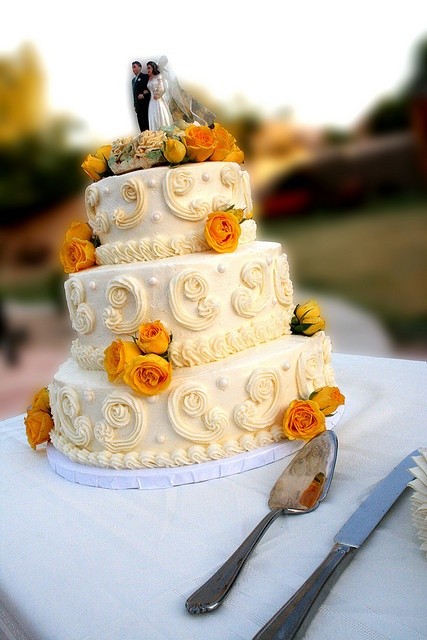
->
[23,54,345,471]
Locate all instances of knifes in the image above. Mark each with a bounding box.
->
[253,446,423,640]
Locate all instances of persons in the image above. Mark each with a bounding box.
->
[131,60,150,132]
[142,61,174,129]
